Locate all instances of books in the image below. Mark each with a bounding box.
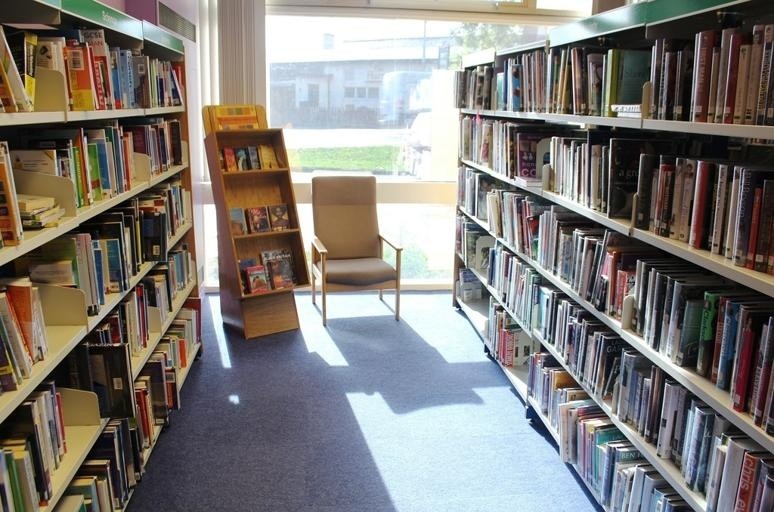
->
[219,145,298,294]
[0,25,199,512]
[455,25,774,511]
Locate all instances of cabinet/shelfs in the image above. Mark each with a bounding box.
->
[1,0,202,512]
[204,128,310,340]
[454,0,774,512]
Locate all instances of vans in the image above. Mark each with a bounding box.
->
[394,68,459,176]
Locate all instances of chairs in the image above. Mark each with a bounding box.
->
[309,175,403,327]
